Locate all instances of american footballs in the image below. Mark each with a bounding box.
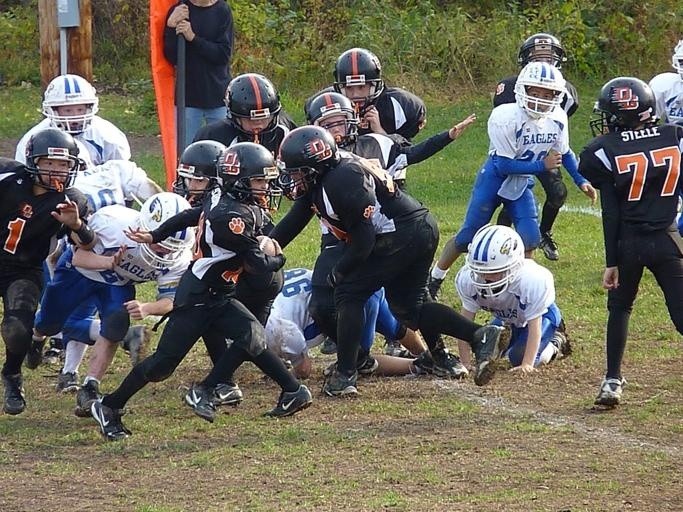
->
[245,236,276,273]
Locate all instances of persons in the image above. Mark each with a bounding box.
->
[647,39,683,240]
[428,61,597,298]
[492,33,579,262]
[1,48,511,442]
[576,77,682,408]
[457,223,571,376]
[162,0,234,151]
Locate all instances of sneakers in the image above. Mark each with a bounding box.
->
[120,325,152,366]
[593,379,624,406]
[556,320,570,361]
[216,382,244,403]
[538,229,559,261]
[262,385,313,417]
[43,338,64,364]
[315,319,511,400]
[184,387,216,424]
[55,367,79,393]
[4,373,26,414]
[90,395,132,440]
[26,337,47,369]
[427,266,445,300]
[75,381,103,416]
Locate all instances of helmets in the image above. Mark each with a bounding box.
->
[42,75,97,106]
[309,92,361,147]
[277,125,340,200]
[26,128,79,161]
[596,77,659,130]
[518,34,568,69]
[513,61,566,95]
[75,138,93,168]
[464,221,525,272]
[217,142,284,210]
[171,140,227,206]
[670,37,683,60]
[333,48,384,103]
[222,73,280,143]
[138,192,196,269]
